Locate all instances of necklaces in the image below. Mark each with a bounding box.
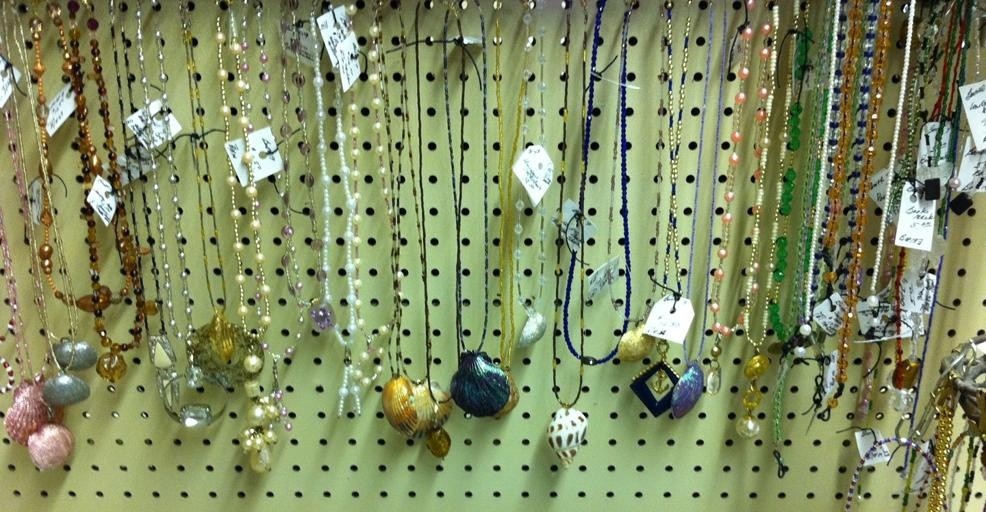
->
[0,2,982,511]
